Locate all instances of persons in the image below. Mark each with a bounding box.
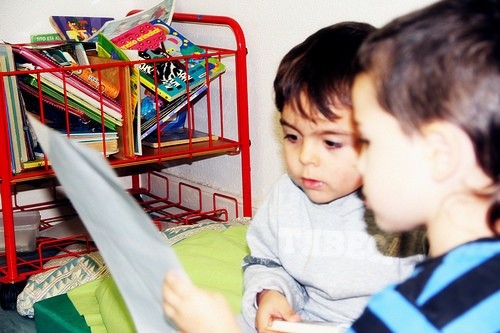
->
[160,0,500,332]
[239,15,430,331]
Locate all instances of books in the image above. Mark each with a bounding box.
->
[2,0,225,180]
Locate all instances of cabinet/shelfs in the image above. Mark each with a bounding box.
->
[0,9,252,311]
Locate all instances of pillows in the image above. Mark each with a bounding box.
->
[66,219,251,332]
[15,215,251,319]
